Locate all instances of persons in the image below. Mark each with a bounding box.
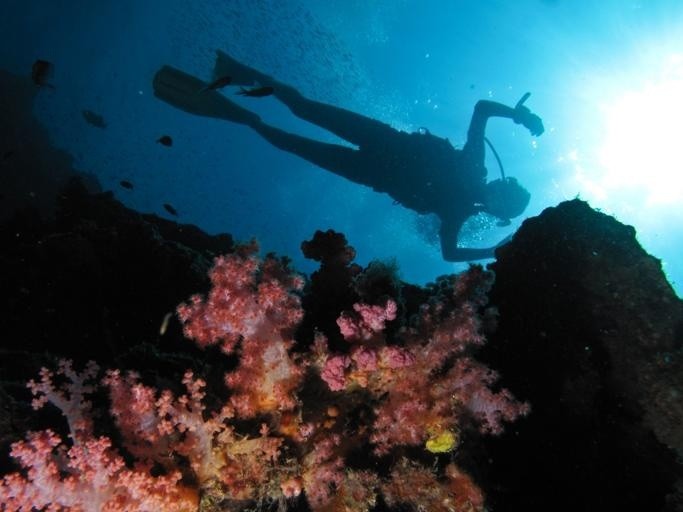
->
[153,50,544,262]
